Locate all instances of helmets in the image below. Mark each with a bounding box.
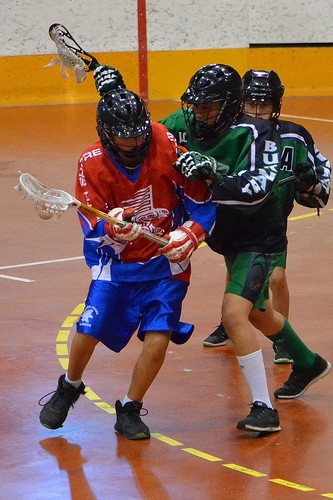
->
[240,69,284,118]
[96,87,153,167]
[179,63,245,142]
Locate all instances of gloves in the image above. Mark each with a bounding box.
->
[93,65,127,96]
[158,220,207,267]
[105,207,144,244]
[175,151,231,183]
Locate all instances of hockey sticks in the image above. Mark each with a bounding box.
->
[278,175,295,184]
[49,24,212,187]
[18,173,171,246]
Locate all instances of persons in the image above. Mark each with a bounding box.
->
[92,64,332,433]
[39,87,218,440]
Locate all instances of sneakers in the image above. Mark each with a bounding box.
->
[235,401,282,432]
[203,323,231,347]
[113,397,153,440]
[38,374,86,430]
[273,353,331,399]
[272,342,293,363]
